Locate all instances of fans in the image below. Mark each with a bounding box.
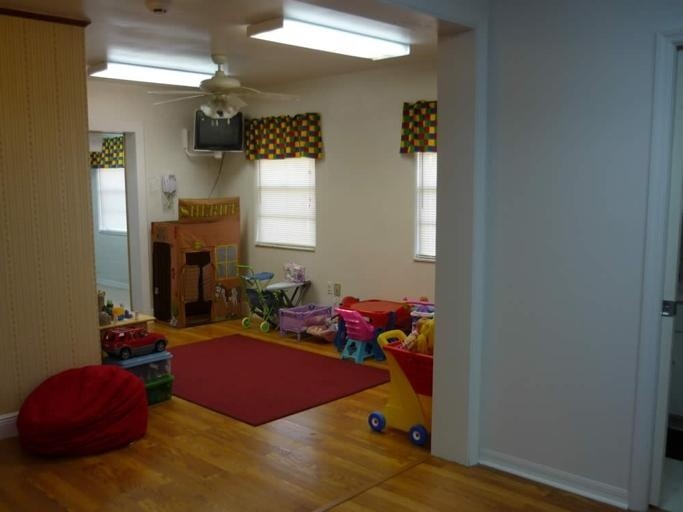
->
[143,53,309,106]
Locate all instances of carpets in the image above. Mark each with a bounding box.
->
[162,333,391,429]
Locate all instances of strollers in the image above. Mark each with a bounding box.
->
[234,261,287,333]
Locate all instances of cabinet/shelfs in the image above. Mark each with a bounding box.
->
[150,195,243,328]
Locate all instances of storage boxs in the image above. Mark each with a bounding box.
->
[107,350,173,381]
[143,374,172,404]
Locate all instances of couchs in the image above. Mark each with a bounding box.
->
[17,360,149,462]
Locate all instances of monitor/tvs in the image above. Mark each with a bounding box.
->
[194,109,243,153]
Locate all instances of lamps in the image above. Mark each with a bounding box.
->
[200,96,246,123]
[244,14,412,62]
[87,58,212,89]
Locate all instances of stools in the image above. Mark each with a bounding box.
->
[265,281,304,308]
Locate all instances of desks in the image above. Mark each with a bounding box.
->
[99,308,155,333]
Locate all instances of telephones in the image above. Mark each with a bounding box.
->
[162,174,176,193]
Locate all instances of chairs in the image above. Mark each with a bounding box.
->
[333,295,412,364]
